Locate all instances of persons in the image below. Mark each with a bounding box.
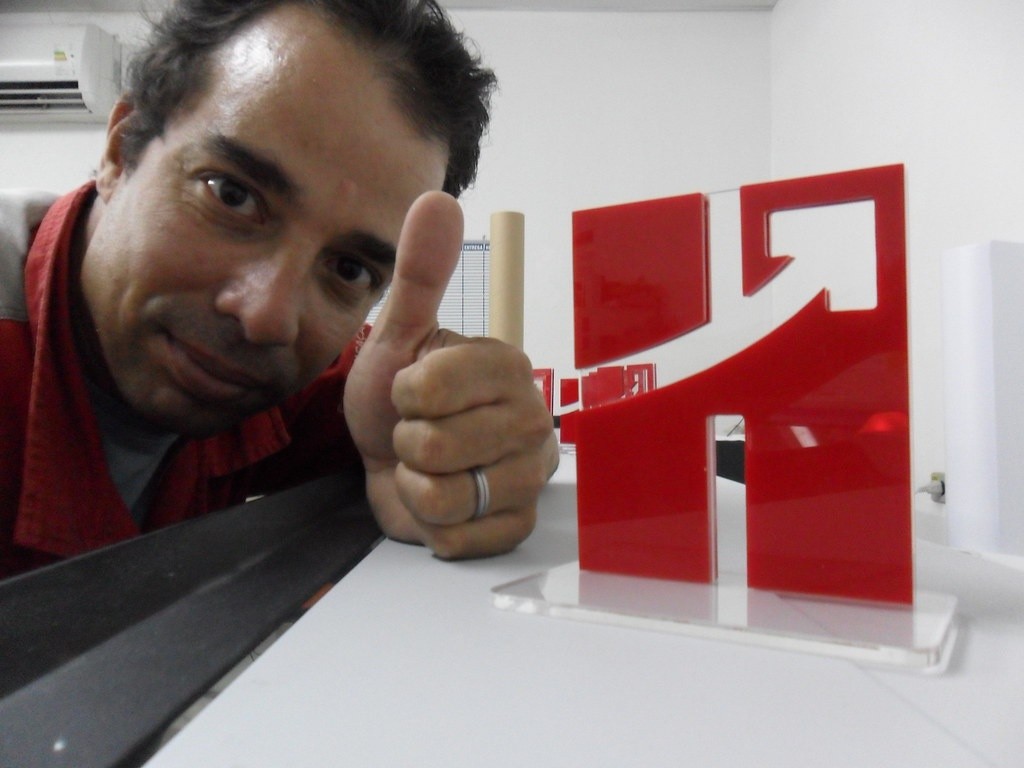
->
[1,1,560,580]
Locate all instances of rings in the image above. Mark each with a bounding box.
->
[471,467,490,521]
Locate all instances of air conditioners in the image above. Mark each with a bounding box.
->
[0,24,119,124]
